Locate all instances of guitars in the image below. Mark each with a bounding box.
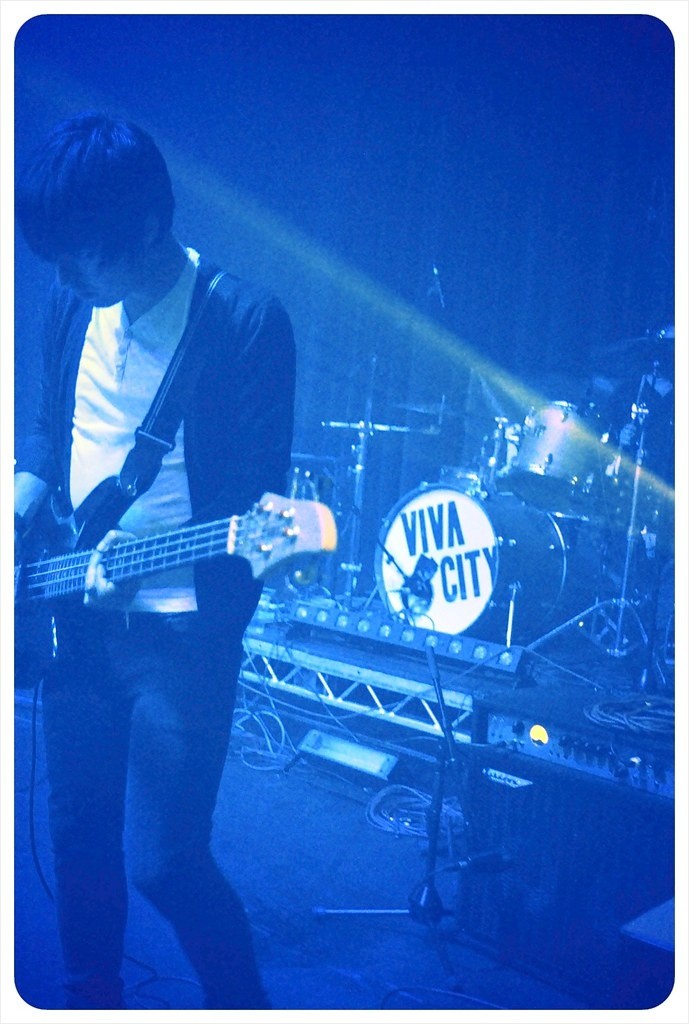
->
[15,493,336,603]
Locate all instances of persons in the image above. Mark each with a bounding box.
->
[14,107,294,1010]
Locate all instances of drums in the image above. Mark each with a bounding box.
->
[513,401,611,507]
[377,481,569,641]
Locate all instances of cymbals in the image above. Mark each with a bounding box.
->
[405,402,465,420]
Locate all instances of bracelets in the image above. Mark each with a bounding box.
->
[13,511,26,536]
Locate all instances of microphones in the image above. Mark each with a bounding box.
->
[424,644,442,681]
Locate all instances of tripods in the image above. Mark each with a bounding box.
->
[309,680,512,996]
